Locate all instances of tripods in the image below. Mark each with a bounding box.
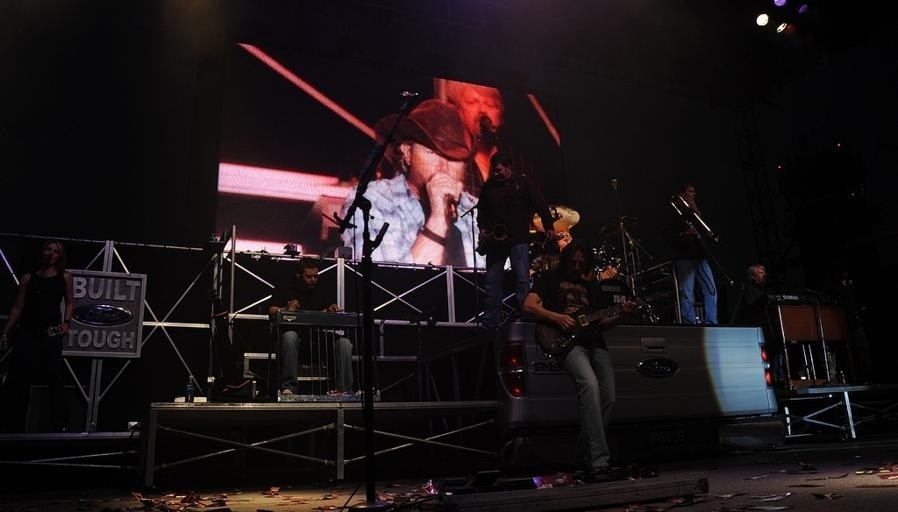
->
[617,220,660,325]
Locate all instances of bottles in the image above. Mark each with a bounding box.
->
[185,375,194,403]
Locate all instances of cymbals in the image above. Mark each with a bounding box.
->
[533,204,581,235]
[616,216,638,227]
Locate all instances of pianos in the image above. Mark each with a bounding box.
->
[270,308,381,402]
[766,293,847,391]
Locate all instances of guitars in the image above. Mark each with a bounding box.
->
[536,300,657,354]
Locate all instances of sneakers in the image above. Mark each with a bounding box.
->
[574,455,637,482]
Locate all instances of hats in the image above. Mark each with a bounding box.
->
[376,99,476,160]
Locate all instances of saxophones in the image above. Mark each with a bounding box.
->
[668,196,720,243]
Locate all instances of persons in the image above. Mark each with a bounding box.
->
[742,265,785,364]
[673,183,719,324]
[523,245,617,483]
[342,99,514,271]
[269,257,354,395]
[476,152,555,324]
[0,240,75,434]
[439,80,504,193]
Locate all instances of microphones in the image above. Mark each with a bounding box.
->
[447,199,458,223]
[611,178,618,192]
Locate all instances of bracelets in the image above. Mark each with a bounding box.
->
[1,333,6,337]
[64,320,70,328]
